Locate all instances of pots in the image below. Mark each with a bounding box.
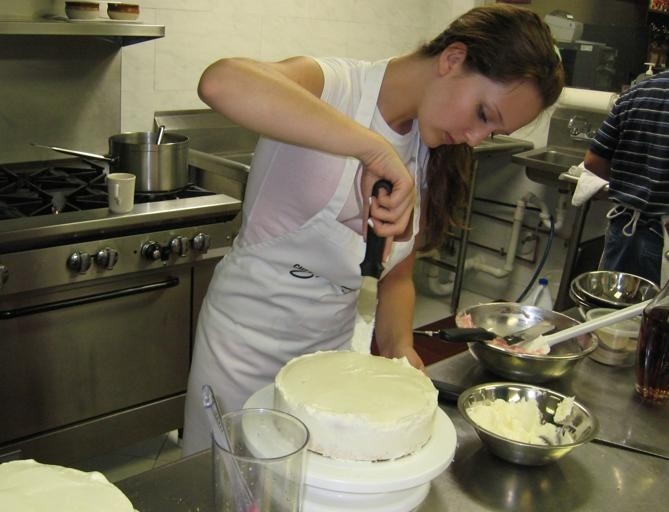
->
[30,131,190,191]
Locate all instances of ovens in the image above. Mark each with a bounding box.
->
[0,270,213,467]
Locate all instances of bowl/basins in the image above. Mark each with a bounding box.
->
[107,1,140,20]
[455,302,599,383]
[457,382,601,467]
[64,1,100,20]
[568,269,660,308]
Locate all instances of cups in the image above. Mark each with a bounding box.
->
[106,172,136,214]
[210,408,310,511]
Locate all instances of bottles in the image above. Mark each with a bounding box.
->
[634,268,669,408]
[530,278,554,311]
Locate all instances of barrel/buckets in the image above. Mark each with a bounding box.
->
[523,278,553,313]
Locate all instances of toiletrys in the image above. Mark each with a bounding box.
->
[642,61,655,79]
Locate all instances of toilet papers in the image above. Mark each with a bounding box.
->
[559,87,619,116]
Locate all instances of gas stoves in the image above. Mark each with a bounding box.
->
[0,155,244,295]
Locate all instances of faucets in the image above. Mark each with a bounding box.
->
[565,115,601,146]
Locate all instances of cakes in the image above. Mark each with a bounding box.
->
[273,348,439,462]
[0,459,135,512]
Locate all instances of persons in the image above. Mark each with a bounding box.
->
[583,46,669,297]
[177,1,565,484]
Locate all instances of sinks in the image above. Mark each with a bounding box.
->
[510,144,616,202]
